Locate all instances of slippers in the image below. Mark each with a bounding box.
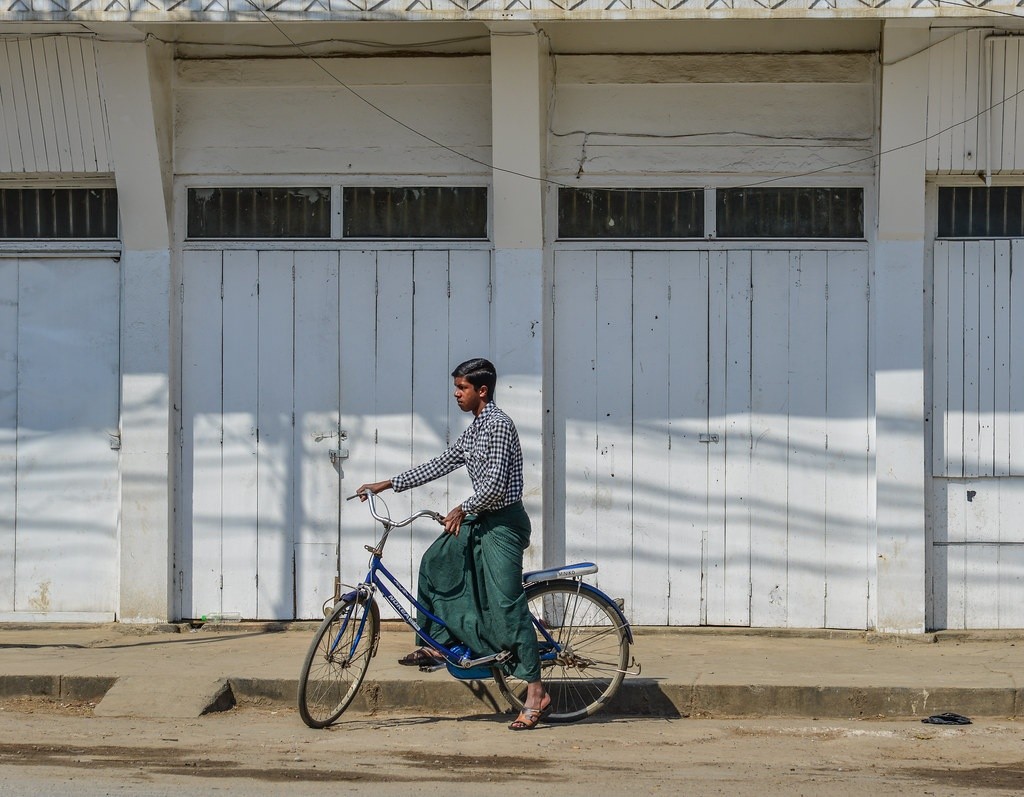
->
[398,647,446,666]
[507,699,553,730]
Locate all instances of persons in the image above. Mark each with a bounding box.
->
[350,356,550,735]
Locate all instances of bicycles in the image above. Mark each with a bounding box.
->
[296,488,644,730]
